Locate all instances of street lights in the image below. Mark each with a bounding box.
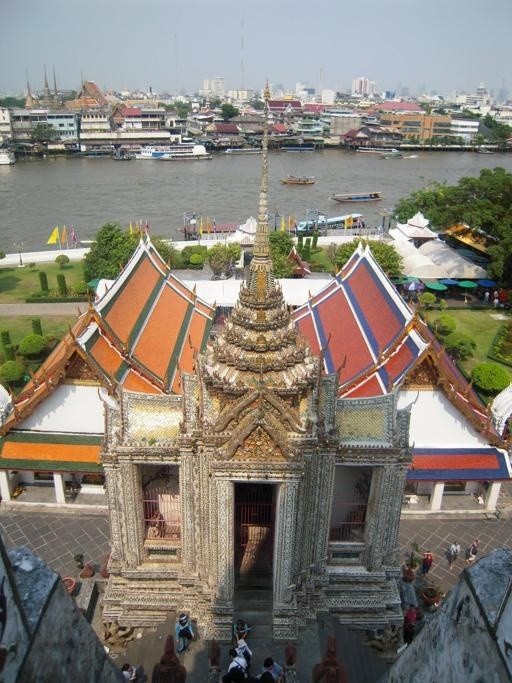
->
[12,240,26,268]
[220,228,232,249]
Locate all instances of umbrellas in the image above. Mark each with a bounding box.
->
[393,278,496,302]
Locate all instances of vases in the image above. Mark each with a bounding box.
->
[62,577,75,593]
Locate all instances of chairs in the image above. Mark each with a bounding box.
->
[402,497,410,509]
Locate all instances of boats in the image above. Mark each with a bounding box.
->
[330,190,382,203]
[278,142,317,152]
[0,146,17,165]
[77,236,173,246]
[73,142,212,160]
[223,146,262,154]
[280,175,319,184]
[284,212,366,234]
[479,148,494,154]
[380,151,403,158]
[356,145,398,155]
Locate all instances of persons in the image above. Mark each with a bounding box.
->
[481,286,507,307]
[405,603,417,624]
[420,537,481,575]
[119,661,137,682]
[231,619,253,641]
[175,613,196,656]
[235,638,253,668]
[226,649,249,676]
[258,657,284,683]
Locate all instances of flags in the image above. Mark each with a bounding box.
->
[343,216,362,237]
[197,217,216,241]
[280,216,298,237]
[128,219,149,240]
[45,222,78,246]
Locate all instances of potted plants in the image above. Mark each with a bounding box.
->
[403,542,425,581]
[421,582,440,606]
[74,554,94,577]
